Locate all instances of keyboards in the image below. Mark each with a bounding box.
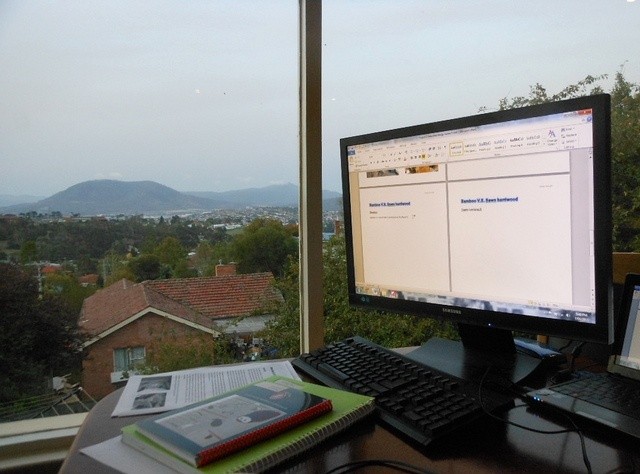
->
[292,335,510,457]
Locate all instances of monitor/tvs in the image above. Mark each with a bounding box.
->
[340,92,614,393]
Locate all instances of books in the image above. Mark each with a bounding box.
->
[134,380,333,468]
[120,375,376,474]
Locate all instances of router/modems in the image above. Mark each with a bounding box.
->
[513,337,563,372]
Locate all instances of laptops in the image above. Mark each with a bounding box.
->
[527,272,640,437]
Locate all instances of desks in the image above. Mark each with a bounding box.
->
[57,251,640,473]
